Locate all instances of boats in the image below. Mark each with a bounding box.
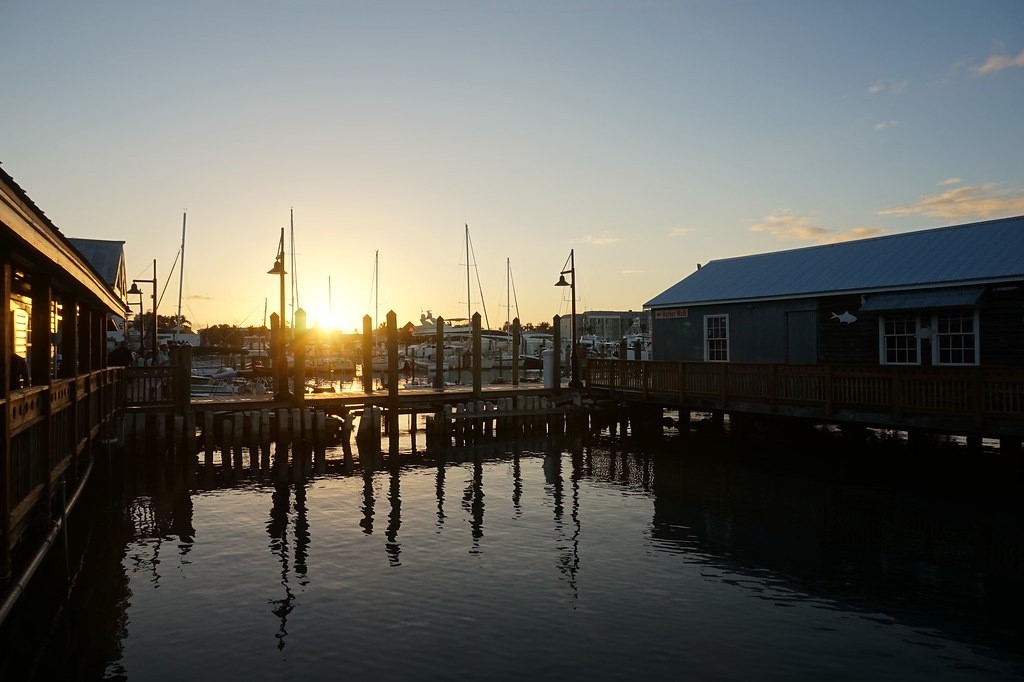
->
[188,224,651,398]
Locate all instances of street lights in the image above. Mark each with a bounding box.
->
[554,248,584,388]
[125,288,146,358]
[126,258,157,365]
[266,226,294,401]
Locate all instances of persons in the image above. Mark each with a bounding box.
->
[110,341,187,402]
[9,350,29,392]
